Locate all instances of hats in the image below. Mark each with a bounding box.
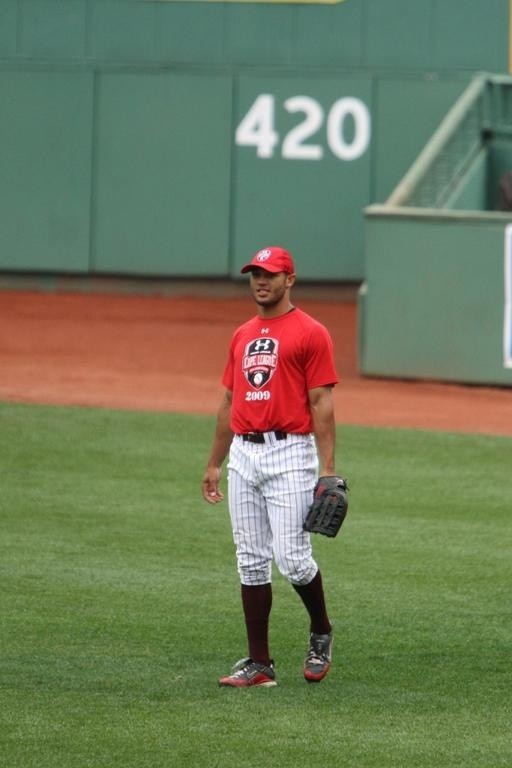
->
[240,246,295,274]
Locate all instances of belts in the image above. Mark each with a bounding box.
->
[235,430,286,443]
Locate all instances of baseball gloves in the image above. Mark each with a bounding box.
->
[301,475,349,538]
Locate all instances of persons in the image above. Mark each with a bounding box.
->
[201,246,350,687]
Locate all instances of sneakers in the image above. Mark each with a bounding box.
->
[218,658,277,688]
[302,634,333,682]
